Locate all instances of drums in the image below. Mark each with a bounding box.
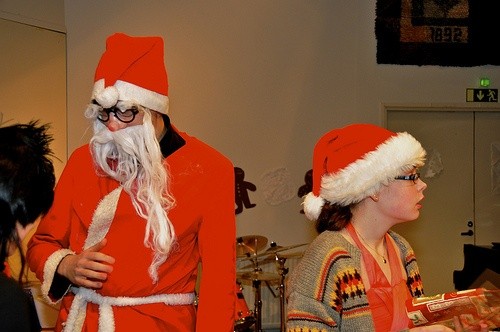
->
[234,281,256,330]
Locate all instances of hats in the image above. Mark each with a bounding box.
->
[90,32,169,115]
[300,123,427,221]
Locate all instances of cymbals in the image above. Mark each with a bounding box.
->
[236,271,280,281]
[237,235,268,260]
[246,241,310,261]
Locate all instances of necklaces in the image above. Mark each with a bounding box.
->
[354,228,388,266]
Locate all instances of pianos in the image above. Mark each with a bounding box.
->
[452,242,500,292]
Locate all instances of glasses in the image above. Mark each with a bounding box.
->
[95,105,139,123]
[391,172,420,185]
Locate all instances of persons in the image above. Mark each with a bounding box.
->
[284,121,492,332]
[1,124,56,331]
[24,34,238,332]
